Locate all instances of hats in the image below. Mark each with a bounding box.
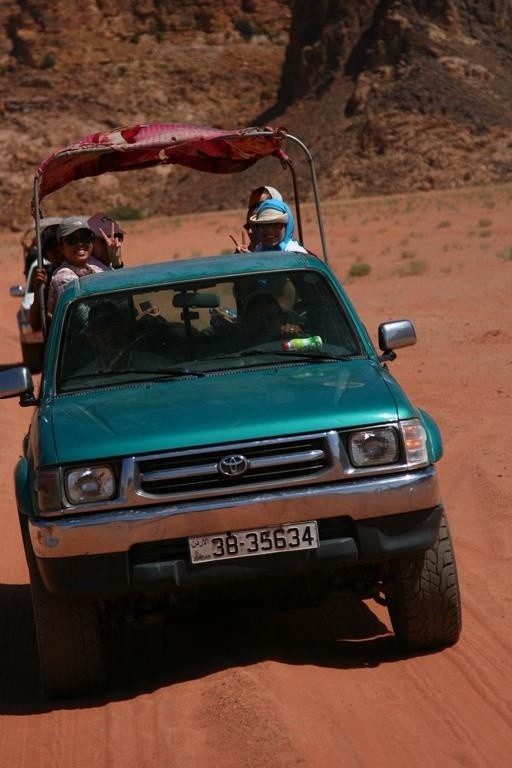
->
[250,204,291,227]
[242,201,264,229]
[86,300,124,332]
[55,216,96,240]
[40,223,60,247]
[86,212,127,238]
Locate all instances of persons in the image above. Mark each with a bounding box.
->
[242,202,273,254]
[200,275,332,356]
[60,301,172,380]
[246,185,284,210]
[207,196,312,326]
[20,215,64,324]
[28,215,163,341]
[84,212,125,269]
[24,223,62,332]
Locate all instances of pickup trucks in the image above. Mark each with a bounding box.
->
[0,250,463,700]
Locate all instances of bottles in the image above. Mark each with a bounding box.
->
[283,336,323,352]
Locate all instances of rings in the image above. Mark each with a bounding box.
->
[289,327,295,331]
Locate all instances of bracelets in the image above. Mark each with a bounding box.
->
[111,260,124,269]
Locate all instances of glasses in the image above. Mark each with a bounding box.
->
[60,233,92,245]
